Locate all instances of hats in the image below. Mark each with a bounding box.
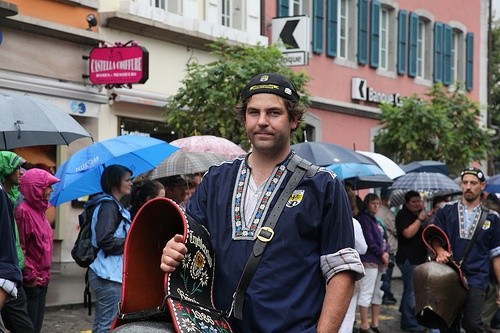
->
[242,72,300,103]
[461,167,484,182]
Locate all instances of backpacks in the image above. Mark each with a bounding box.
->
[71,203,122,268]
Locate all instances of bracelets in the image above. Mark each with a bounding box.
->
[417,217,423,223]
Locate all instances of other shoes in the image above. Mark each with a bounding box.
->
[384,295,397,305]
[370,326,382,333]
[360,328,374,333]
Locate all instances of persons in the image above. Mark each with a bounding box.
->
[0,150,500,333]
[158,73,367,333]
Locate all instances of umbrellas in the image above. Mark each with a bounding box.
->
[0,93,92,153]
[387,171,463,195]
[47,135,182,210]
[291,130,375,168]
[343,174,394,191]
[355,150,406,180]
[325,162,386,182]
[482,173,500,193]
[131,135,248,184]
[453,173,489,186]
[401,160,449,176]
